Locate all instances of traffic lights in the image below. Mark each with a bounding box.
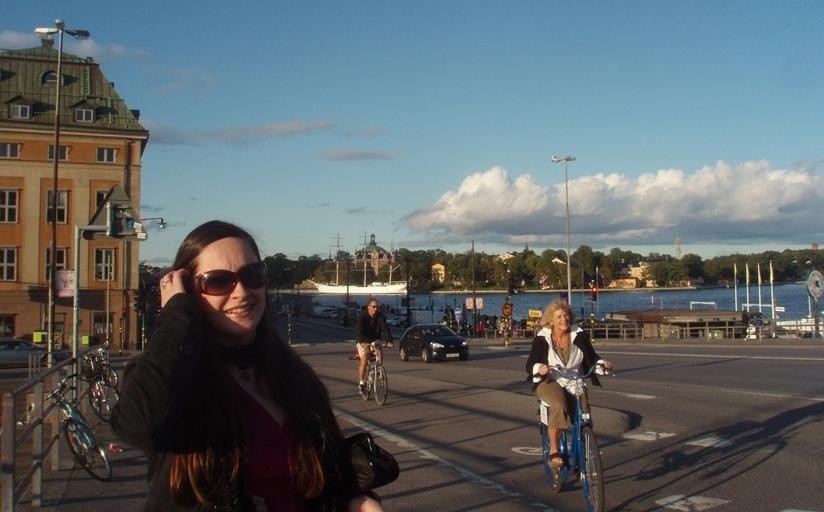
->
[592,286,597,302]
[133,288,143,312]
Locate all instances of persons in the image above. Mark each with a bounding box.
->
[109,219,402,512]
[355,298,392,391]
[522,299,616,468]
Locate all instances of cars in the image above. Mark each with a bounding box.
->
[398,324,469,362]
[0,338,68,367]
[316,309,405,326]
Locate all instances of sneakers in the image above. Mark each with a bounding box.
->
[548,454,564,468]
[359,383,367,393]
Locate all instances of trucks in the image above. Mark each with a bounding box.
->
[410,311,449,324]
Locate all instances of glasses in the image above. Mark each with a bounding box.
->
[190,262,269,296]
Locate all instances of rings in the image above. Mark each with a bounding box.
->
[160,285,164,288]
[162,279,170,287]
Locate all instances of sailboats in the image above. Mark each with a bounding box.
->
[306,231,418,294]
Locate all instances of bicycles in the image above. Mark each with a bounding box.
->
[42,347,120,482]
[355,342,392,406]
[536,362,615,512]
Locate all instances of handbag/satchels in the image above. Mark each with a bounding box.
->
[315,432,401,512]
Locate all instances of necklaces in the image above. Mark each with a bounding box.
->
[555,332,568,364]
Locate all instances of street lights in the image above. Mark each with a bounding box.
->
[121,217,167,348]
[552,154,576,304]
[506,267,511,303]
[30,16,89,366]
